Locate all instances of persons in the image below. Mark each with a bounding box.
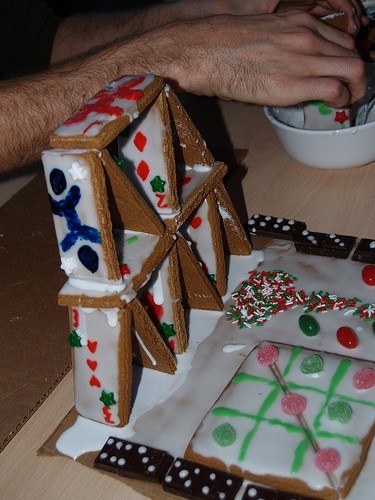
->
[1,0,368,179]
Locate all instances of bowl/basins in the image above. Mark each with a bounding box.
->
[263,85,375,169]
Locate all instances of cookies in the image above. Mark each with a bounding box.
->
[41,74,256,428]
[305,91,375,132]
[184,338,374,499]
